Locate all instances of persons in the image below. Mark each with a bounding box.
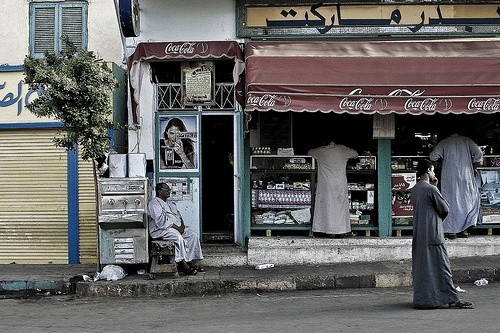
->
[308,139,358,237]
[427,130,484,239]
[410,159,473,309]
[159,118,194,168]
[148,183,206,275]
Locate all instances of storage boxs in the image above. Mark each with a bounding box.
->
[391,174,417,190]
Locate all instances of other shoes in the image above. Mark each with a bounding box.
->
[459,232,468,237]
[183,266,205,275]
[445,233,457,239]
[449,300,475,309]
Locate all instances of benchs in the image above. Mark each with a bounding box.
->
[149,240,181,279]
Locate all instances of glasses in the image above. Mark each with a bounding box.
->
[161,187,172,192]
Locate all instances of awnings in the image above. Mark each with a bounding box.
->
[247,41,500,113]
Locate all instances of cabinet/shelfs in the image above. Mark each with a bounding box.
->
[250,154,379,237]
[391,155,500,236]
